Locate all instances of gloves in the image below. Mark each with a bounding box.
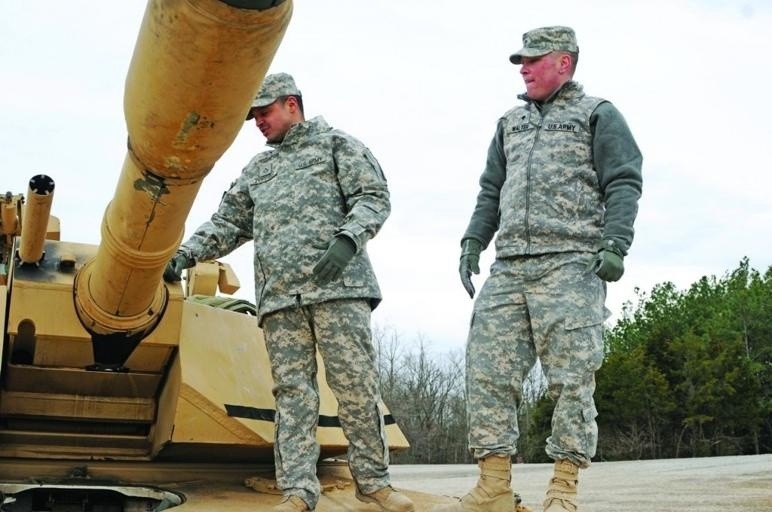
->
[459,239,485,299]
[163,250,194,283]
[586,245,625,281]
[312,235,355,283]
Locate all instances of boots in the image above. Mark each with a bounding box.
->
[544,457,579,512]
[431,454,516,511]
[356,484,415,512]
[272,494,317,511]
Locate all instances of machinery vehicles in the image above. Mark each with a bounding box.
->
[1,0,534,511]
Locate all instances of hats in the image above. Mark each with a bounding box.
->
[509,26,579,64]
[246,72,301,121]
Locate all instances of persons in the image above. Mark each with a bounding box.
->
[162,71,418,512]
[432,25,643,512]
